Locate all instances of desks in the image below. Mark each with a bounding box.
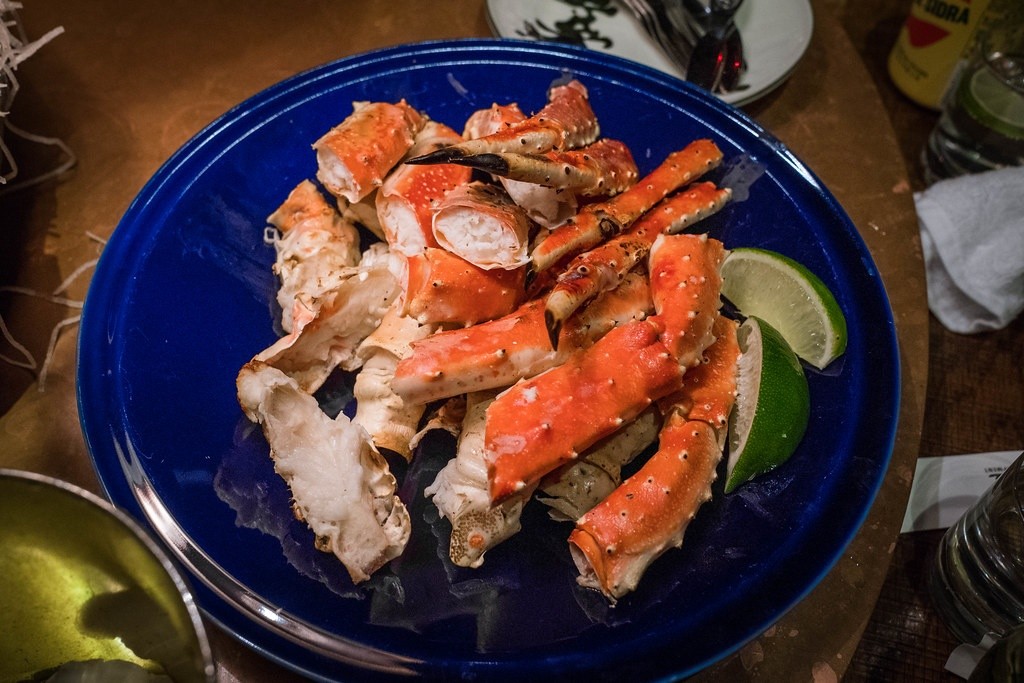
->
[0,0,929,683]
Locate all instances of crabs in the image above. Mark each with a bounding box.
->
[232,79,745,607]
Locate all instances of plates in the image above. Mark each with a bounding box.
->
[0,466,218,683]
[483,0,815,107]
[73,37,903,683]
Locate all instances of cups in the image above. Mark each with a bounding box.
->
[887,0,1024,188]
[936,446,1024,642]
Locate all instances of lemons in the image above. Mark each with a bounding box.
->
[722,315,809,494]
[720,247,849,371]
